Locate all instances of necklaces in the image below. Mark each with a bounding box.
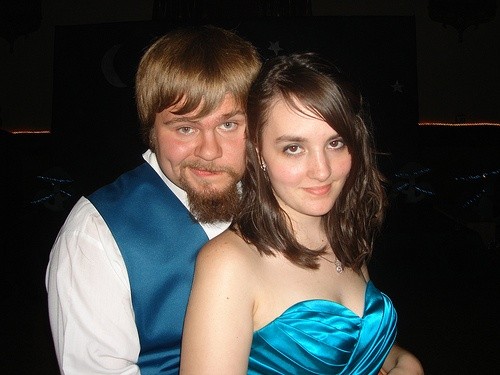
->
[313,250,344,274]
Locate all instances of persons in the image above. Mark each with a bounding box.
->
[177,48,399,374]
[43,24,426,375]
